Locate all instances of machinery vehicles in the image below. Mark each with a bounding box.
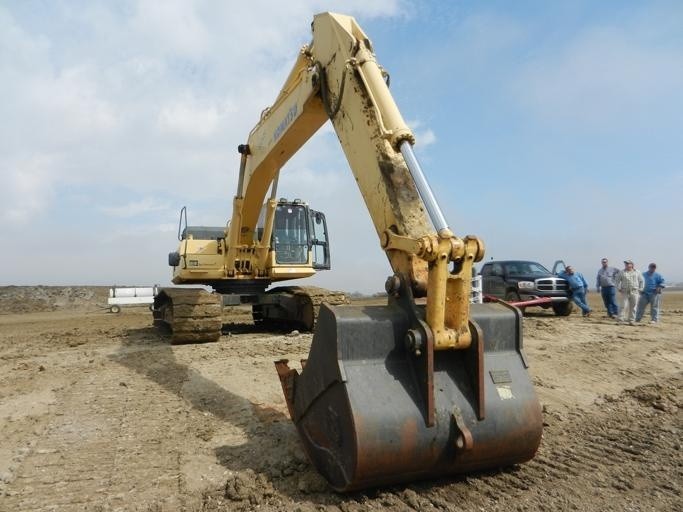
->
[147,12,546,493]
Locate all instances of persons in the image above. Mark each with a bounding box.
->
[557,266,593,317]
[594,258,620,318]
[614,259,645,326]
[634,263,665,324]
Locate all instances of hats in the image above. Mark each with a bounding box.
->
[624,260,633,264]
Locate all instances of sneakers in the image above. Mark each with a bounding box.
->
[583,309,592,318]
[610,315,655,325]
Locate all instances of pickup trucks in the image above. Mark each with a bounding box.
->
[472,258,573,316]
